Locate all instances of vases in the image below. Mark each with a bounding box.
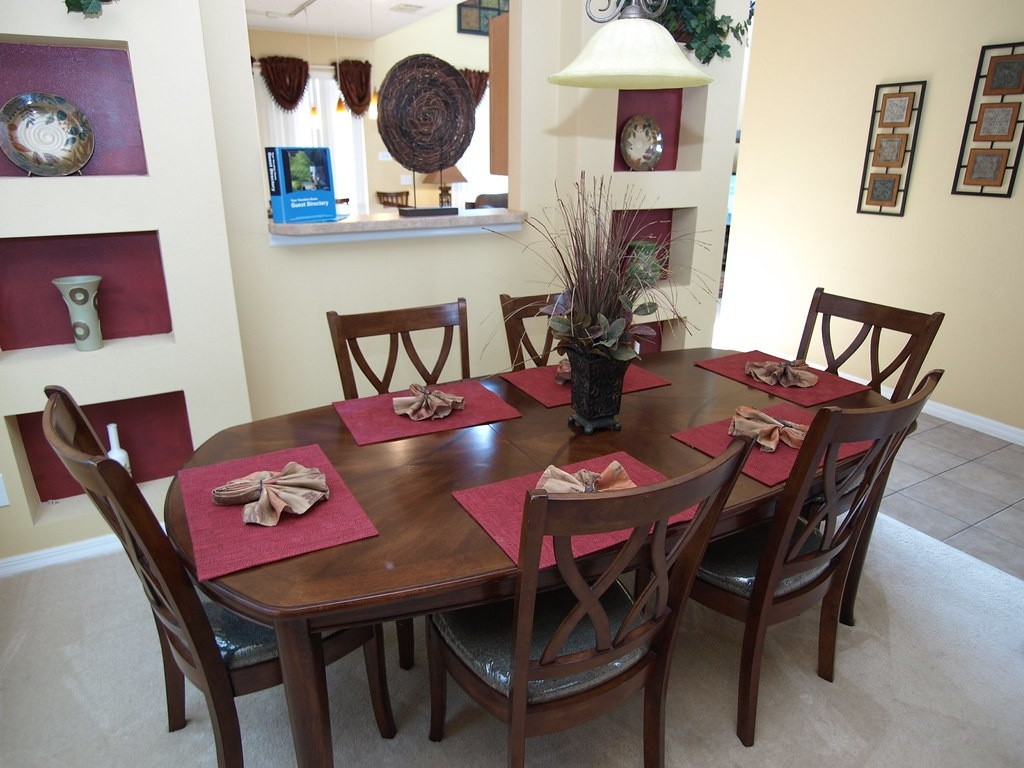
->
[53,276,102,351]
[105,423,131,475]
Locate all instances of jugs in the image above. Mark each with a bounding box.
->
[623,241,668,290]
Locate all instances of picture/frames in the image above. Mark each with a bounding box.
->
[879,91,915,128]
[964,148,1009,186]
[866,173,900,207]
[872,134,908,168]
[973,102,1021,142]
[983,54,1024,96]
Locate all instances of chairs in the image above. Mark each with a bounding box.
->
[39,387,397,767]
[425,432,760,768]
[326,301,471,667]
[633,368,946,746]
[794,287,946,525]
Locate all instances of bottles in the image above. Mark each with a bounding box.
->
[104,422,133,480]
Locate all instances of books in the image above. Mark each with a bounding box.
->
[264,147,338,226]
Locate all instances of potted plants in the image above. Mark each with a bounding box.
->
[489,170,720,437]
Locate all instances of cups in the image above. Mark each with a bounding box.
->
[51,274,103,352]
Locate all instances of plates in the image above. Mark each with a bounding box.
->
[0,91,95,176]
[620,113,663,171]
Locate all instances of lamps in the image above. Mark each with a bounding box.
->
[425,165,467,207]
[548,0,714,90]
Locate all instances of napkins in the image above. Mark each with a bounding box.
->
[554,357,572,385]
[210,462,330,526]
[393,384,465,421]
[728,407,808,454]
[745,358,819,388]
[533,461,638,492]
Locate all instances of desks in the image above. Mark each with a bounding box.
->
[162,345,918,768]
